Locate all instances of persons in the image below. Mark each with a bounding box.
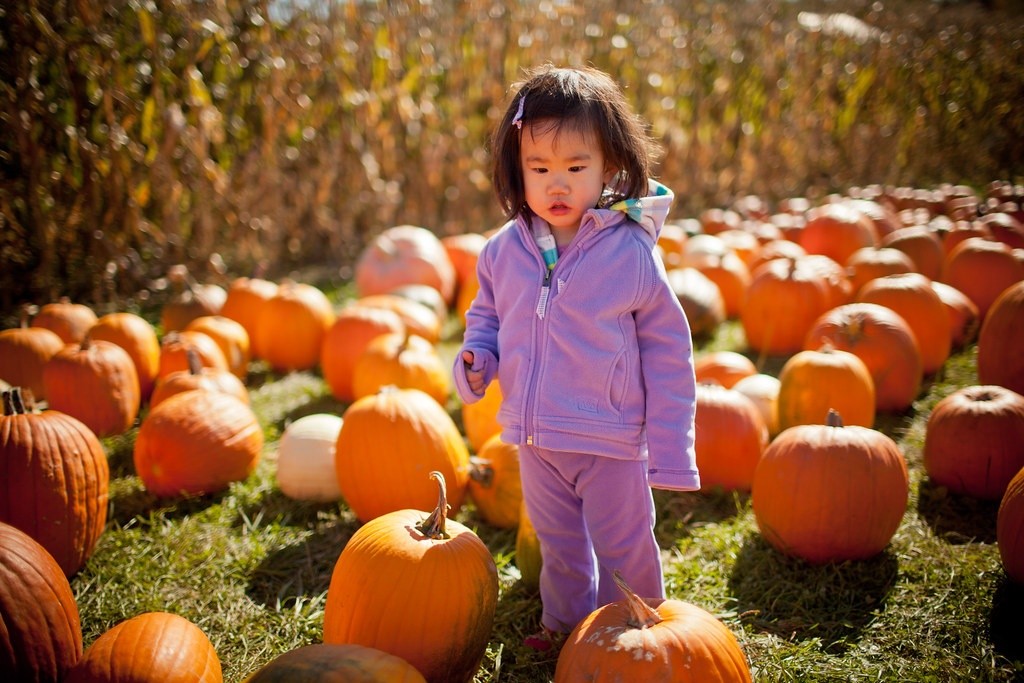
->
[452,67,702,652]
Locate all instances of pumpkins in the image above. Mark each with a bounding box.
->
[0,181,1024,683]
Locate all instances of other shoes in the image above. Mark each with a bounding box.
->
[524,627,571,651]
